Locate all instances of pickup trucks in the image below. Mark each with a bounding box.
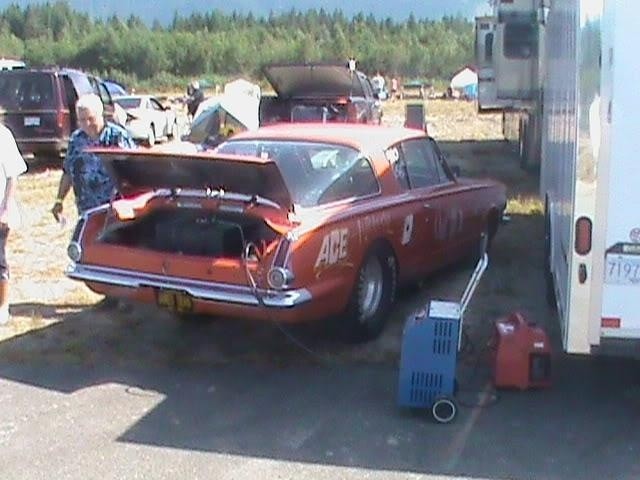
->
[251,58,387,128]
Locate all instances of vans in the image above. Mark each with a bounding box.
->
[0,66,117,163]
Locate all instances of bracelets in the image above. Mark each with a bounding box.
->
[55,198,63,203]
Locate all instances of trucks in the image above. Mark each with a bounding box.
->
[491,0,640,366]
[475,0,543,171]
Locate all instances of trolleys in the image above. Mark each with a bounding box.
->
[389,226,491,429]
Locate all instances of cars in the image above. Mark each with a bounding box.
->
[110,91,182,149]
[62,121,514,342]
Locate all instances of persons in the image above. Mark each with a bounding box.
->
[371,68,437,103]
[186,80,204,131]
[53,93,138,311]
[0,122,27,324]
